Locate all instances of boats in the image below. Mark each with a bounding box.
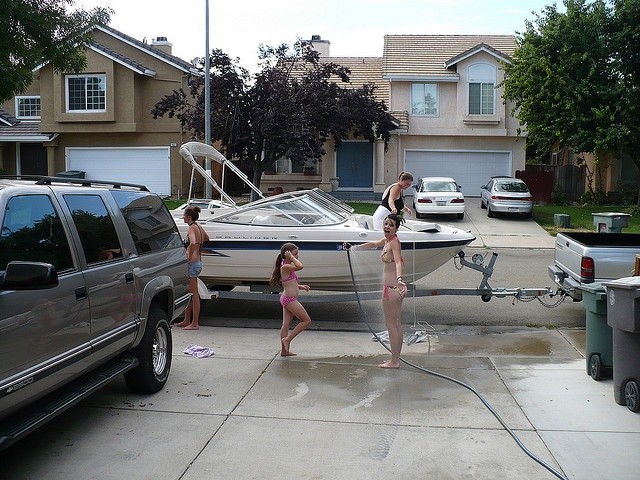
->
[167,141,475,291]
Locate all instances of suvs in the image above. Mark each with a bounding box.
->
[1,175,193,453]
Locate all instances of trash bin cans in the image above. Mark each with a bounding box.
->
[56,171,86,184]
[601,275,640,412]
[579,281,614,379]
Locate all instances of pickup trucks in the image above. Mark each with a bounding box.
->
[548,232,640,302]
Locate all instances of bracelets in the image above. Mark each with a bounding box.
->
[397,277,403,281]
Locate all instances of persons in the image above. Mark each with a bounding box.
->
[371,172,413,231]
[173,206,209,331]
[343,214,409,369]
[269,242,311,357]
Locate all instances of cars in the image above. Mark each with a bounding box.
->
[481,175,533,218]
[411,177,465,219]
